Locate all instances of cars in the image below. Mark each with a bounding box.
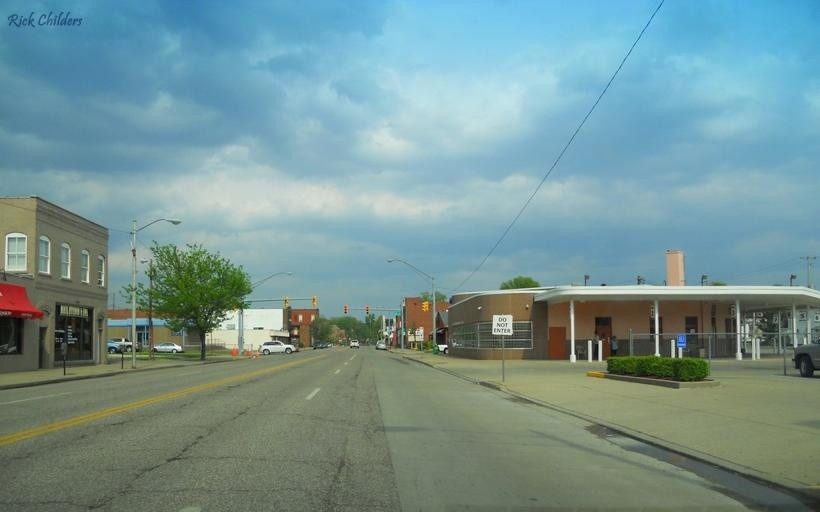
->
[258,340,296,355]
[107,339,126,355]
[792,336,820,378]
[3,328,91,354]
[350,339,360,348]
[803,325,820,344]
[313,339,327,349]
[151,341,184,354]
[375,339,386,350]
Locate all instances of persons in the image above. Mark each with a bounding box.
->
[611,335,618,356]
[592,330,599,359]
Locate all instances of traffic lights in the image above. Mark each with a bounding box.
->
[425,301,428,311]
[344,304,348,314]
[422,302,425,312]
[365,305,370,315]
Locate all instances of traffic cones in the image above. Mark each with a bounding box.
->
[232,348,236,357]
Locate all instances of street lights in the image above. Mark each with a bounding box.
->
[131,216,182,369]
[237,271,293,357]
[386,257,436,354]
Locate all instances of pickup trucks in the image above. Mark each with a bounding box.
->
[438,340,462,354]
[110,336,142,353]
